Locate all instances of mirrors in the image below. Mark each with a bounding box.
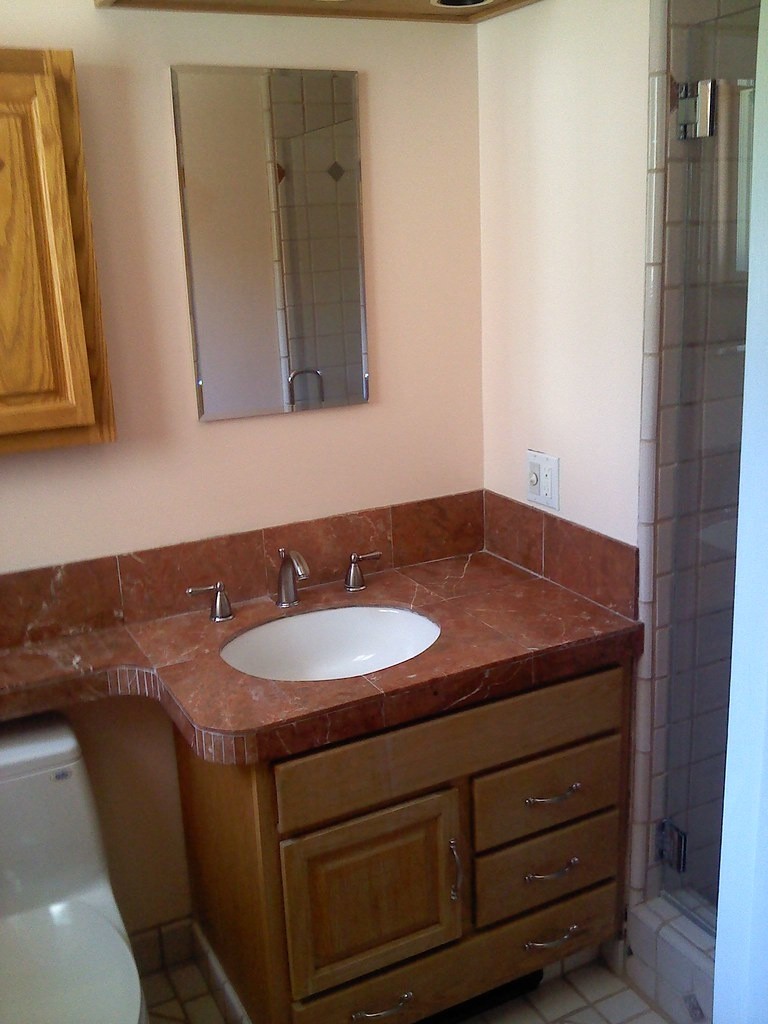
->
[168,65,370,423]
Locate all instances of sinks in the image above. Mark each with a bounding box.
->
[220,604,440,681]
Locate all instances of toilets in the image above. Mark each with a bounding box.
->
[1,714,149,1024]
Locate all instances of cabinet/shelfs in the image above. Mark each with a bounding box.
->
[0,48,118,453]
[171,660,638,1024]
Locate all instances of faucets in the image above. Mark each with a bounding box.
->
[274,549,310,608]
[286,369,324,412]
